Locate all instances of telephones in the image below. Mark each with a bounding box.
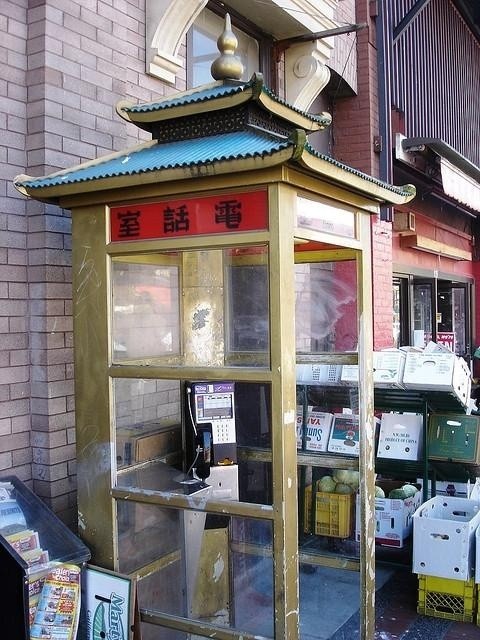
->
[179,375,240,476]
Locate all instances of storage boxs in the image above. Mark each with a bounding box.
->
[403,348,471,408]
[341,350,405,388]
[314,492,353,538]
[290,362,342,386]
[328,412,380,457]
[376,412,425,462]
[428,412,480,465]
[296,410,333,451]
[417,573,474,624]
[409,495,480,581]
[356,481,423,549]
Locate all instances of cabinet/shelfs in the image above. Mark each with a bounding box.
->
[0,474,91,640]
